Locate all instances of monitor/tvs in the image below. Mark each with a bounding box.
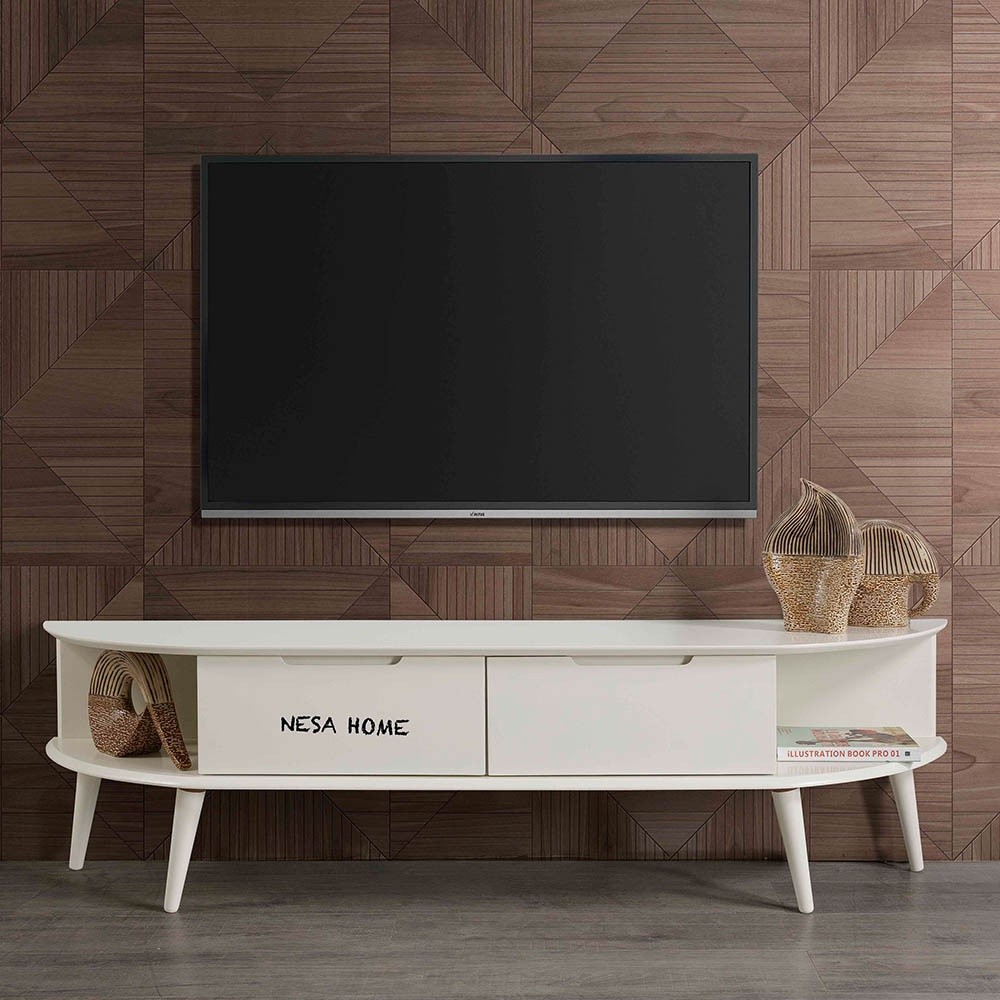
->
[200,152,758,519]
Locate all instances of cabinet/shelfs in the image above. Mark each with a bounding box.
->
[41,620,952,913]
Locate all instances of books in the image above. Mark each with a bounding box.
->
[776,726,922,762]
